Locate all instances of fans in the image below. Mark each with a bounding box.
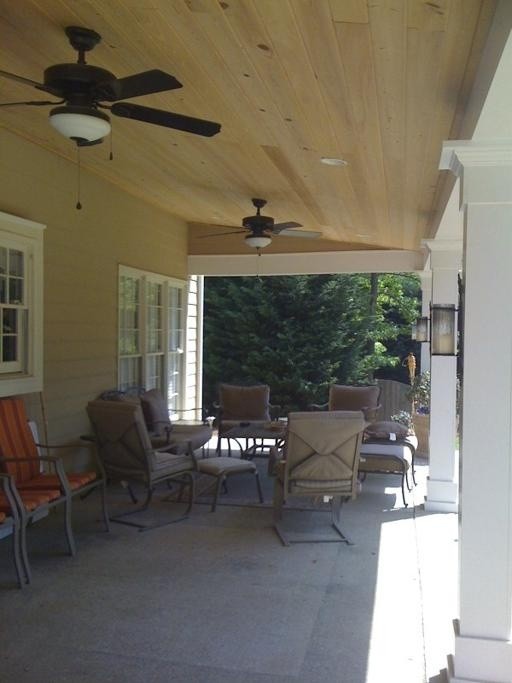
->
[1,24,222,209]
[196,197,322,283]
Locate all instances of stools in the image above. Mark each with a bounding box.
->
[177,457,263,512]
[359,429,420,507]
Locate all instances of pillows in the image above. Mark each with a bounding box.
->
[366,420,415,439]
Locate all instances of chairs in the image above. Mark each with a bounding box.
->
[3,396,111,585]
[329,384,382,420]
[268,410,364,546]
[80,388,212,533]
[214,385,280,458]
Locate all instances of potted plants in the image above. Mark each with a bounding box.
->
[408,369,461,457]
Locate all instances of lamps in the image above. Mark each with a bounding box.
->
[48,103,113,148]
[416,273,465,356]
[243,227,274,250]
